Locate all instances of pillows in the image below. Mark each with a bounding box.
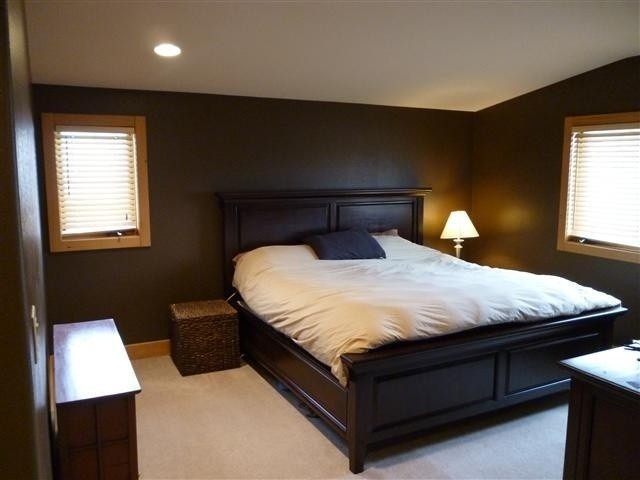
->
[303,225,388,261]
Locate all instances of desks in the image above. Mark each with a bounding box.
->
[167,299,240,378]
[554,346,639,479]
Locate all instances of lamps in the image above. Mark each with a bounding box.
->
[439,209,481,258]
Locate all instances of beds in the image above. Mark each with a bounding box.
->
[217,186,628,474]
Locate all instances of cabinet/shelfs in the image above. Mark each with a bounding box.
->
[48,315,143,478]
